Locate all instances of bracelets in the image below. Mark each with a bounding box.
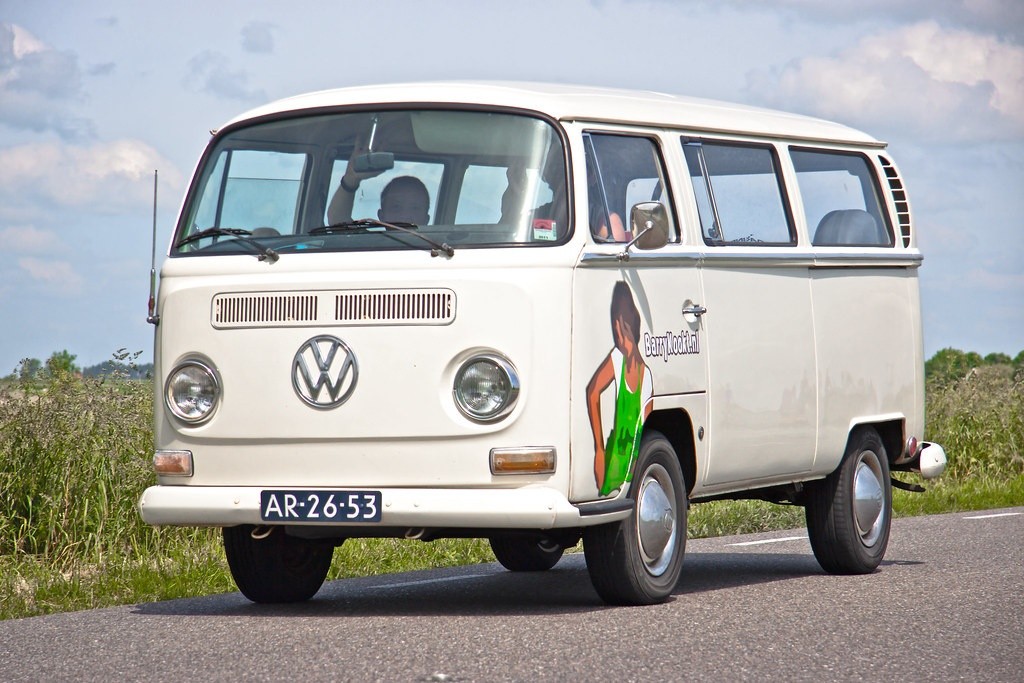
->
[339,176,361,193]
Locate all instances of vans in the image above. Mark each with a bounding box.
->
[138,80,948,606]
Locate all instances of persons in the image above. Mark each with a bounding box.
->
[246,126,431,239]
[514,125,629,242]
